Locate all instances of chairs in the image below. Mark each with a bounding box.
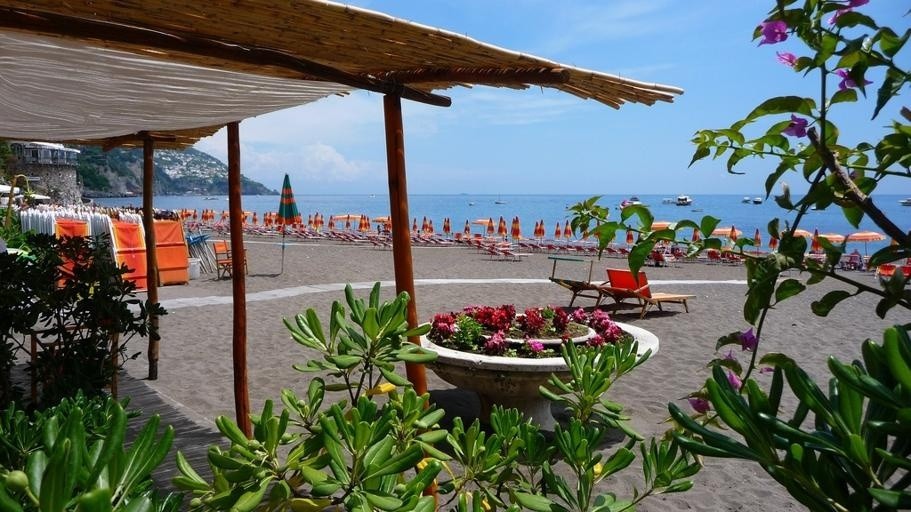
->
[546,254,612,310]
[704,248,865,272]
[212,240,248,279]
[596,267,698,321]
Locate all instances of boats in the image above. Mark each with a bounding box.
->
[742,197,752,203]
[753,198,762,205]
[469,202,474,206]
[898,197,911,205]
[494,201,506,205]
[811,207,817,210]
[621,194,705,213]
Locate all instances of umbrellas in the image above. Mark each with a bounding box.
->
[275,168,304,225]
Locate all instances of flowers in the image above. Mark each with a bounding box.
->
[426,303,626,360]
